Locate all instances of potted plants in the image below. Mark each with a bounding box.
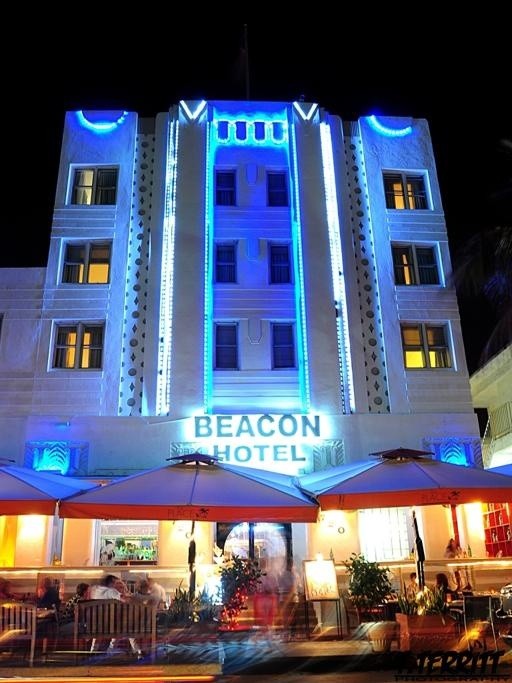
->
[341,552,399,653]
[394,580,461,653]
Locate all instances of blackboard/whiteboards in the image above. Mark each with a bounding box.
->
[303,559,340,601]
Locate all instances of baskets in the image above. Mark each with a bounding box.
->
[366,621,396,652]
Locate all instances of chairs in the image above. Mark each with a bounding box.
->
[342,584,512,654]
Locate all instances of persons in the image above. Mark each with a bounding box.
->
[61,575,166,660]
[433,573,448,614]
[37,577,61,655]
[406,571,418,613]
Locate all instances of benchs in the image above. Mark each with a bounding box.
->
[1,599,156,664]
